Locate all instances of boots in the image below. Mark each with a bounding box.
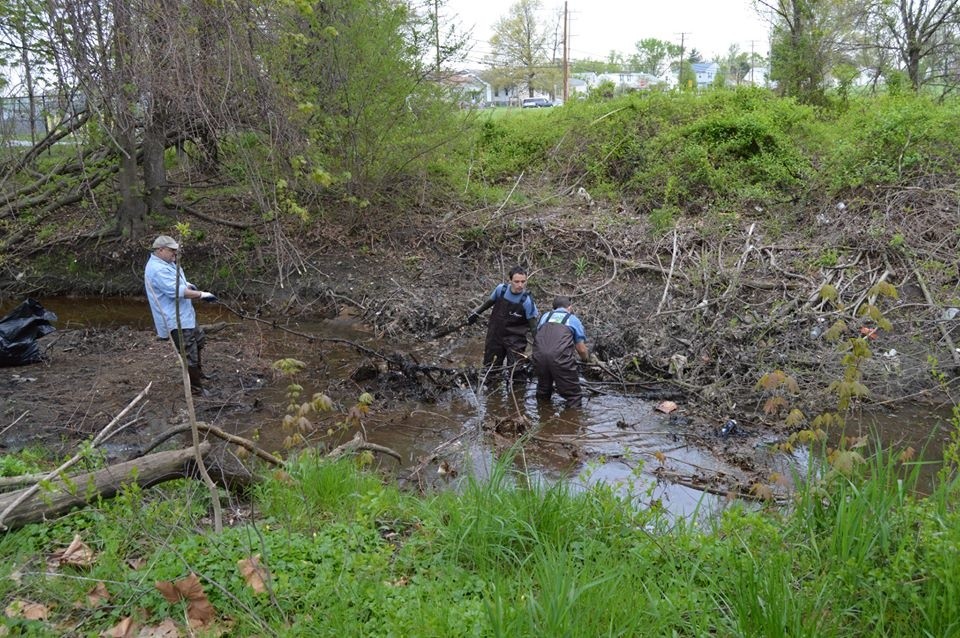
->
[189,366,210,398]
[199,364,216,380]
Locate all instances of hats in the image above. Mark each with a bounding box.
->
[152,235,179,249]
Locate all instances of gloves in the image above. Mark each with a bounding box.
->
[468,312,478,324]
[187,280,198,291]
[199,291,218,303]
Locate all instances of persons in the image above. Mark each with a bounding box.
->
[532,296,589,405]
[145,236,215,389]
[468,267,539,380]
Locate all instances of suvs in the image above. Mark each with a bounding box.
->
[522,97,553,108]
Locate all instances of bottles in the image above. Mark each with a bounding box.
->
[810,317,825,340]
[722,419,736,436]
